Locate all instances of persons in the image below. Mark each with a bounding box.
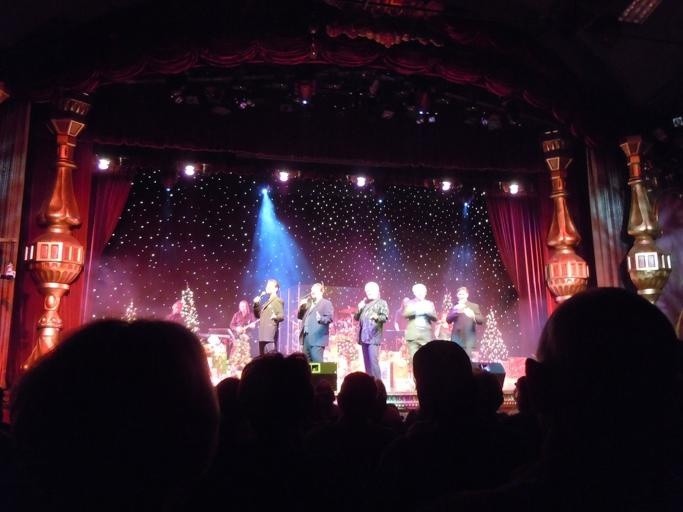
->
[296,283,334,363]
[353,281,390,380]
[252,279,283,356]
[401,283,437,390]
[446,286,484,360]
[0,288,683,511]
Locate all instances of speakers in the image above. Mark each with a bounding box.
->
[308,361,337,392]
[470,363,506,390]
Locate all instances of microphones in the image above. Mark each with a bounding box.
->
[253,291,266,306]
[363,297,367,302]
[306,296,311,301]
